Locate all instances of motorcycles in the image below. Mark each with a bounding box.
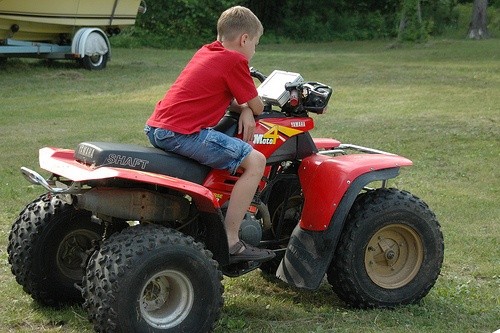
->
[5,66,445,333]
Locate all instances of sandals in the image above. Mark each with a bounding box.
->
[229,240,276,262]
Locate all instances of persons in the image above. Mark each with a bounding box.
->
[143,5,277,264]
